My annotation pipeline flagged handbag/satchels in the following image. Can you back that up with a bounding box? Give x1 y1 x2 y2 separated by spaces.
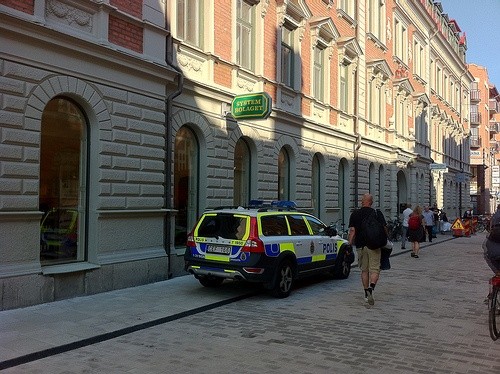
345 245 355 264
380 248 392 270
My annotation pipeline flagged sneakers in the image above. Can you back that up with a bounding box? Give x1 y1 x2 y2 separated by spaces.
366 288 374 305
365 297 368 303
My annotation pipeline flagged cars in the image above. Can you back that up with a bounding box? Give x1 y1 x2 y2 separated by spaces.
184 200 356 298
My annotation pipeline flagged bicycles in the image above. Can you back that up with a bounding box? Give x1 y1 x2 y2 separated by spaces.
476 214 492 232
329 215 402 243
484 271 500 341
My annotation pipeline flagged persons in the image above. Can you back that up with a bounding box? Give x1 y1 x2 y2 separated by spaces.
400 203 500 303
348 194 387 306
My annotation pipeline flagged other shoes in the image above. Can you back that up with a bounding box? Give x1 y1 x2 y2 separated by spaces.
401 247 406 249
411 253 419 258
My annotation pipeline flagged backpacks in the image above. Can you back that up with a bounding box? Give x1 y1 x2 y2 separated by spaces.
408 214 420 230
488 211 500 243
358 209 387 250
